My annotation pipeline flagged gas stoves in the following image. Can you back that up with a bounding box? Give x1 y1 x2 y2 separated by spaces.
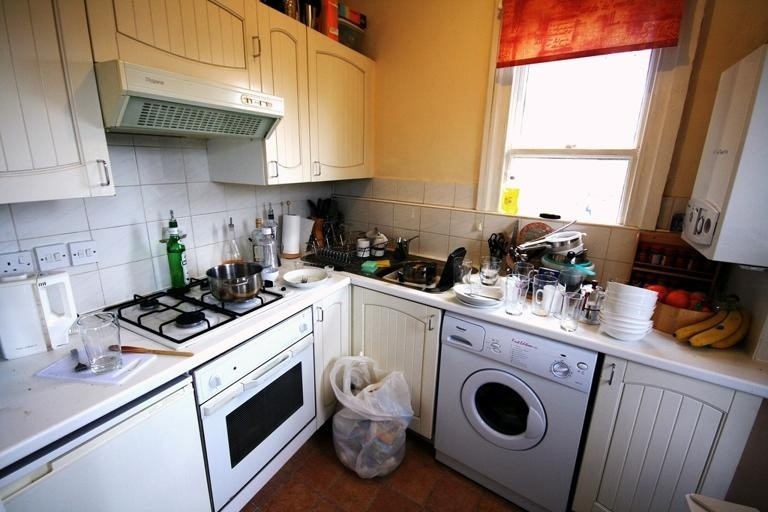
104 275 301 348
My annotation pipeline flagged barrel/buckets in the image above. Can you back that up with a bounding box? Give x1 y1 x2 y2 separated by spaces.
541 254 598 291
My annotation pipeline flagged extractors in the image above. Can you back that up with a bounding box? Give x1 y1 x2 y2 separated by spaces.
90 59 285 145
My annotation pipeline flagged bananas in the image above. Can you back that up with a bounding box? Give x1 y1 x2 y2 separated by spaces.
672 302 751 349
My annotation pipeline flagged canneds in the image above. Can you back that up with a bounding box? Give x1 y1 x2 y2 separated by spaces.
356 238 384 258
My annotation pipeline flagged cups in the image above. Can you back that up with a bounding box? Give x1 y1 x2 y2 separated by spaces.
78 311 122 373
478 256 502 284
371 236 385 256
356 238 369 257
452 255 474 282
508 260 583 331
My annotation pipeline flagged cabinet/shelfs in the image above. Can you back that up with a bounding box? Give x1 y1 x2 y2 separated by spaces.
0 1 116 206
316 284 352 430
206 1 306 186
307 25 374 186
351 279 444 445
84 1 250 88
568 352 768 511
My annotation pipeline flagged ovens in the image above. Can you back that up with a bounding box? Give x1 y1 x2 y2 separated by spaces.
199 332 317 512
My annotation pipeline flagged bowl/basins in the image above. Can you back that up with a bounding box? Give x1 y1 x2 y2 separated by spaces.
261 267 280 283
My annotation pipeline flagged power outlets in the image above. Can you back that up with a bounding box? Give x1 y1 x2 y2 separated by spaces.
0 253 20 278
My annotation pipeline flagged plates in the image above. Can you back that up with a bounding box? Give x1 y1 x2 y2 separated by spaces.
283 268 327 288
598 280 660 342
452 283 504 311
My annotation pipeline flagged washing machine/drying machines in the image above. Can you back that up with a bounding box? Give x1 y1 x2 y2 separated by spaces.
433 310 599 512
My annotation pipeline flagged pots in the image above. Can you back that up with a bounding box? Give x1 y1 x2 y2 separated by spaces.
206 260 267 304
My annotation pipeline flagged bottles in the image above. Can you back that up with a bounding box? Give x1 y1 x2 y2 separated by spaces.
249 202 281 267
165 218 192 290
224 223 242 263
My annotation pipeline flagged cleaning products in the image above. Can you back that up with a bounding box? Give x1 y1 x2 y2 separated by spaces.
500 175 521 215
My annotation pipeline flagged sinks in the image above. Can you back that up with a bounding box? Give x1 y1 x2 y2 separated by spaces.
375 260 479 293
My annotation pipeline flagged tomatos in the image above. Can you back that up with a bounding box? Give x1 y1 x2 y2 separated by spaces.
644 284 713 312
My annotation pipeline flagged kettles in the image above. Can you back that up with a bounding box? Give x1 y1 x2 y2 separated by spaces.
0 271 79 361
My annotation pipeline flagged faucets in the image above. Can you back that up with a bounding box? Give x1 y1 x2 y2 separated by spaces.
395 234 419 257
